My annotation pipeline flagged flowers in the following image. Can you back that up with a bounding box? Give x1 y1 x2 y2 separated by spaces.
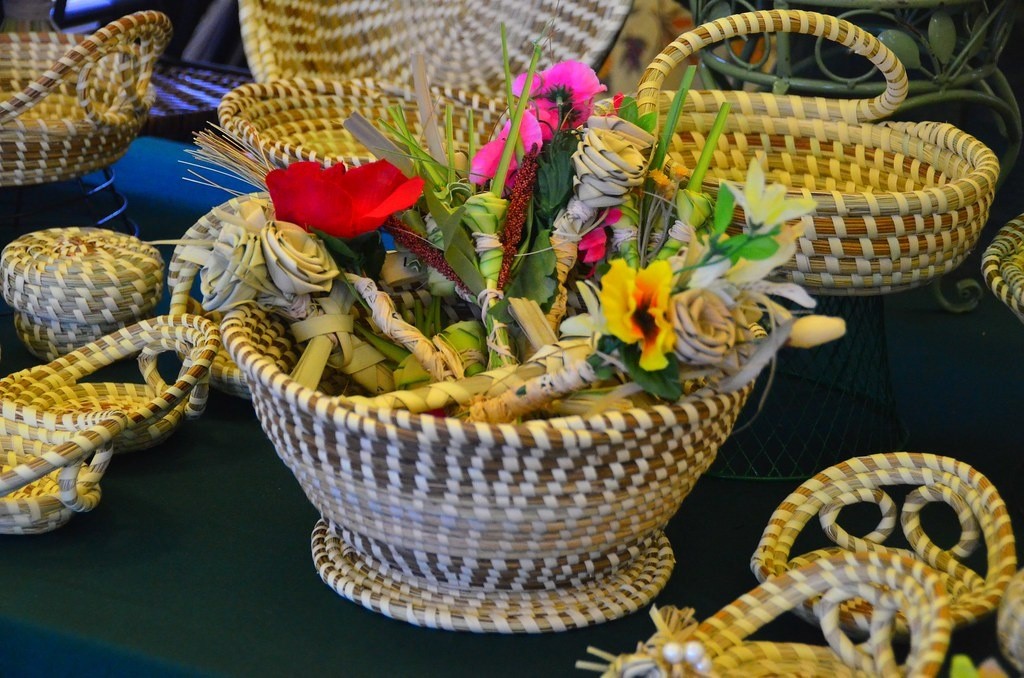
139 18 845 423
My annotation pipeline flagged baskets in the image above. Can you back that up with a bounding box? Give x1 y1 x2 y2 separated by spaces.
591 8 1001 297
0 0 770 633
981 211 1024 323
